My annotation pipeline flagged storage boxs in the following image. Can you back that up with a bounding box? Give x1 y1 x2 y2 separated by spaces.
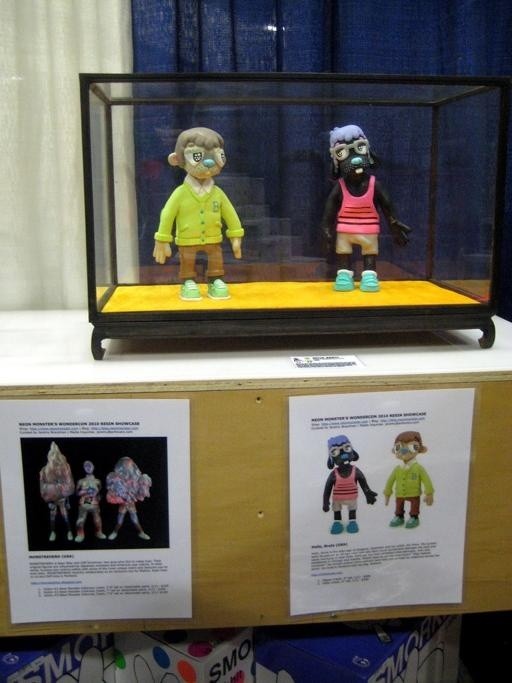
0 613 465 681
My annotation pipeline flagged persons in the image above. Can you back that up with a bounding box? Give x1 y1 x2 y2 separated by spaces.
151 127 245 300
322 125 413 293
